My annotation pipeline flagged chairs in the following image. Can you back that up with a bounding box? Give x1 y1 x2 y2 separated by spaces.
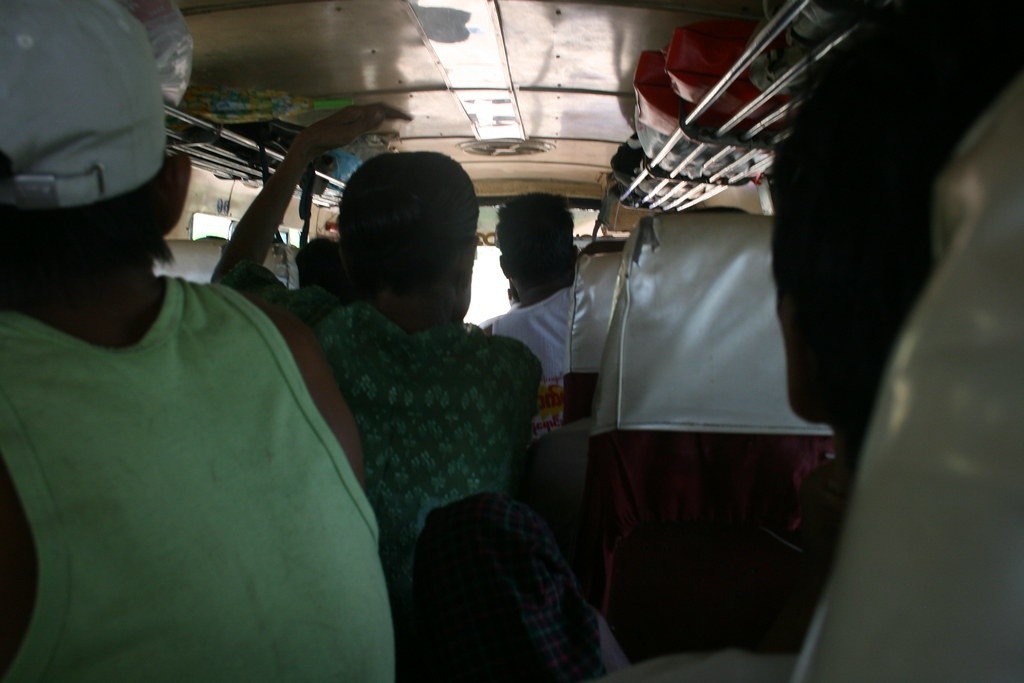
793 82 1024 683
560 250 622 426
570 212 841 664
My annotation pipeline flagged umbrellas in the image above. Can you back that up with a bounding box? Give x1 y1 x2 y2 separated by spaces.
178 70 350 124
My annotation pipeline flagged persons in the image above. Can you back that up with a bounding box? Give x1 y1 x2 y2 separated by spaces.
218 103 543 593
415 0 1023 683
297 235 341 286
0 5 396 682
492 194 578 381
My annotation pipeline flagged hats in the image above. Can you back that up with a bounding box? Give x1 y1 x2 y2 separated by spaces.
0 0 166 212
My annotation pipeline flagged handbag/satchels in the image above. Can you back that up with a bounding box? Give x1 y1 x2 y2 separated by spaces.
138 0 308 180
609 17 799 209
